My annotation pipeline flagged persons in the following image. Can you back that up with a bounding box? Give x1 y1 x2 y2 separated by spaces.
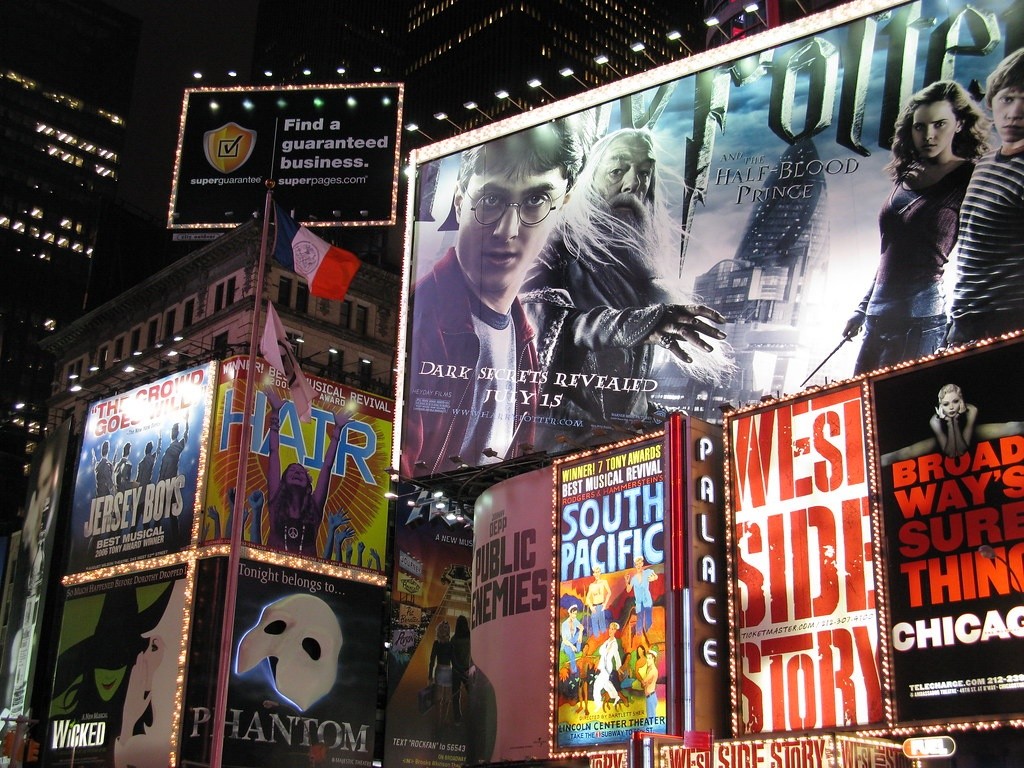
426 615 473 722
86 407 191 565
401 120 726 479
203 486 382 572
841 45 1024 377
260 383 356 558
878 384 1024 470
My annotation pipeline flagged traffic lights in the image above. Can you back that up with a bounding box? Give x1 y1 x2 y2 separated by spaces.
27 738 41 763
2 730 15 757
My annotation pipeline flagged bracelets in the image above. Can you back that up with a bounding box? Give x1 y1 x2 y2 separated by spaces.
270 417 280 432
331 435 339 441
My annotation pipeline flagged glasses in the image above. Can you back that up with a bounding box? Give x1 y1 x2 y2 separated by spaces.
461 183 569 227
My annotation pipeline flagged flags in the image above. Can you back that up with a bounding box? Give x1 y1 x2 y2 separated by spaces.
269 190 361 303
259 302 320 425
